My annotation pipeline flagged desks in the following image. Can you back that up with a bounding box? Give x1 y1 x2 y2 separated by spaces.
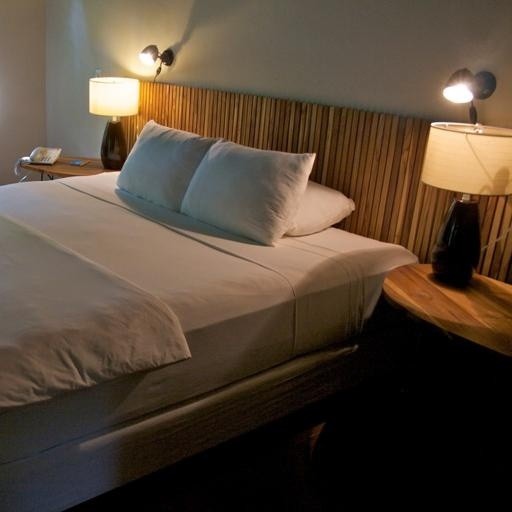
20 155 122 182
381 262 512 359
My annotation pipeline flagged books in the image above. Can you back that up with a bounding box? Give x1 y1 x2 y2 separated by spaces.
68 159 91 168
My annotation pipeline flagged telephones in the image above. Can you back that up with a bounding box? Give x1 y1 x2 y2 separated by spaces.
30 146 62 165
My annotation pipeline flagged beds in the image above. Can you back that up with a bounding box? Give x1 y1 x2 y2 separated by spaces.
0 74 512 512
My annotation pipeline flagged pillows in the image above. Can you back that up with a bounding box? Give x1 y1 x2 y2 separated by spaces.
116 119 219 214
178 136 316 250
283 178 355 238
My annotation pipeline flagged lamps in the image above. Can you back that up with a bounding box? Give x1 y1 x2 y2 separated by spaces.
138 43 174 66
445 67 496 101
420 120 512 288
88 77 140 171
